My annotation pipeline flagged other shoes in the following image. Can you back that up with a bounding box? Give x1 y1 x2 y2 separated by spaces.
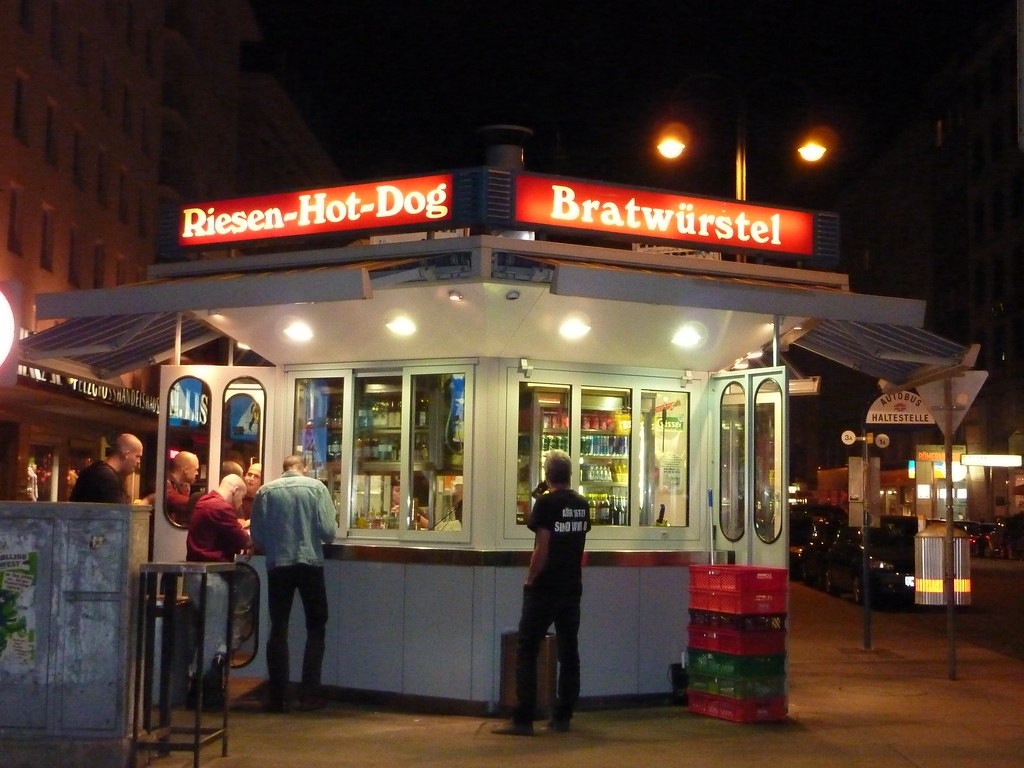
302 695 328 710
264 701 288 712
186 674 198 709
202 670 223 710
549 720 569 730
491 725 534 735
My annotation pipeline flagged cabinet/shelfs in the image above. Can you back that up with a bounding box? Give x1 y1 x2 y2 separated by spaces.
527 386 658 524
305 380 445 471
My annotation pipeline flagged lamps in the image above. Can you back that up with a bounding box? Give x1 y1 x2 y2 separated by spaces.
748 347 764 359
734 360 749 369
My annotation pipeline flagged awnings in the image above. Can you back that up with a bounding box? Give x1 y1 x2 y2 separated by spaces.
14 302 220 385
38 260 419 321
794 316 981 394
519 255 928 328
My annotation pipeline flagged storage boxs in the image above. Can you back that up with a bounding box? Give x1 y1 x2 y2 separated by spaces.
687 673 787 700
688 563 789 593
686 646 788 677
687 607 788 633
686 623 787 655
688 588 789 615
687 690 789 724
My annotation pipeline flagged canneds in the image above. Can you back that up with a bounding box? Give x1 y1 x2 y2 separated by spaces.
581 435 628 456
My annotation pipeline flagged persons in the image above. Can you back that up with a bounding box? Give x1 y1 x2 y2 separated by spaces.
220 461 244 482
69 433 155 506
491 450 592 735
391 476 429 528
249 454 337 713
152 451 199 522
236 464 261 527
181 474 256 714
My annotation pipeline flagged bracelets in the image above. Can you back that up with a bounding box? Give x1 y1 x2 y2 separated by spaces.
416 513 421 523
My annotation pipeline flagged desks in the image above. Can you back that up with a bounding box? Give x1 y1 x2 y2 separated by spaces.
134 560 238 768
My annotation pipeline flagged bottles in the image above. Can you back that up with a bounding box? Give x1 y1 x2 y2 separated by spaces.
581 436 628 456
757 502 775 534
415 435 429 461
372 401 401 428
580 460 626 482
327 409 342 428
531 480 547 499
416 399 429 428
327 435 342 461
583 494 628 526
355 410 371 436
357 508 400 529
358 439 400 461
